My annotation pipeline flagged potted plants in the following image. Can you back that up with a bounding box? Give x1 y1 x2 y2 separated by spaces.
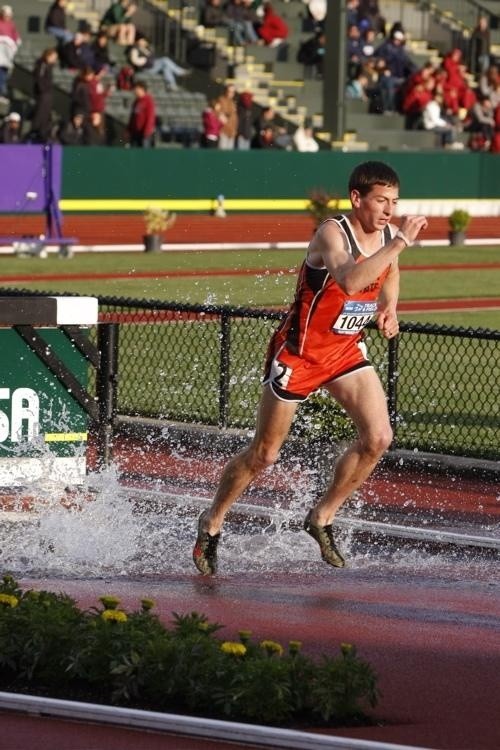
141 205 177 251
448 209 470 245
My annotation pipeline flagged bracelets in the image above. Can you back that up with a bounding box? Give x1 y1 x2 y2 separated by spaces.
396 230 410 247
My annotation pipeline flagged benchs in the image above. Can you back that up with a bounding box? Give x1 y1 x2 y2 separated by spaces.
0 0 499 150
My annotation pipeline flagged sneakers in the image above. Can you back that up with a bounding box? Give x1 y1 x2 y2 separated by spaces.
303 510 346 568
193 509 220 577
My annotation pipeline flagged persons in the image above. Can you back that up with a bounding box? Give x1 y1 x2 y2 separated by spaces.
193 161 429 576
0 1 320 152
295 1 500 152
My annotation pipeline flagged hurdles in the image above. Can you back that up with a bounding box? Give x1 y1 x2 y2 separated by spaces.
0 296 119 493
